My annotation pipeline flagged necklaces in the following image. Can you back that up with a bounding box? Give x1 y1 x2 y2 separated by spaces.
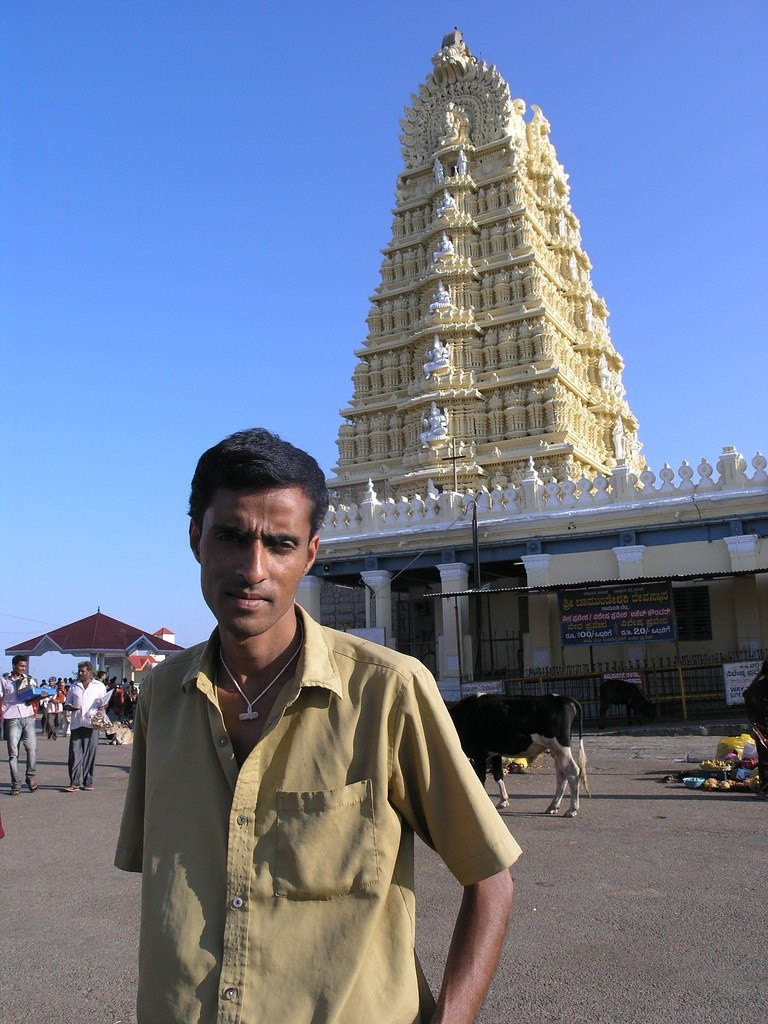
220 617 303 720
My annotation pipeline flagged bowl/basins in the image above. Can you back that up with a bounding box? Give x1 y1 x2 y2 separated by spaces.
705 771 731 780
683 777 705 788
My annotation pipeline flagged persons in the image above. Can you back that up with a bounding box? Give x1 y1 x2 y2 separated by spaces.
551 164 638 460
433 236 455 261
743 660 768 800
437 192 456 217
423 334 451 379
512 98 528 150
429 283 451 314
420 403 449 449
0 655 144 795
113 427 523 1024
433 158 444 182
456 150 467 175
437 110 460 147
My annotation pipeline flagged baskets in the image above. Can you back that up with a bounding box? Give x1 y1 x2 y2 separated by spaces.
683 777 705 789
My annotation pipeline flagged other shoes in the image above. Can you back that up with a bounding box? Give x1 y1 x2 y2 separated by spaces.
53 733 56 740
10 788 20 795
63 784 80 791
26 778 39 792
48 734 51 739
760 791 768 800
84 785 94 790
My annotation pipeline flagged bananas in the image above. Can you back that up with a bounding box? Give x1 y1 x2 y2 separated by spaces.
700 758 735 767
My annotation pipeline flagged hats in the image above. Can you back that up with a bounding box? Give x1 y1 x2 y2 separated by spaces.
48 676 56 682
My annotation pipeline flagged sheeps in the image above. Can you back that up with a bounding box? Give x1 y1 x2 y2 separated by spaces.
105 721 134 746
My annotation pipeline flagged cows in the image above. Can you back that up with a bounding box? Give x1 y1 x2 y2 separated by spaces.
443 692 591 818
597 679 657 733
